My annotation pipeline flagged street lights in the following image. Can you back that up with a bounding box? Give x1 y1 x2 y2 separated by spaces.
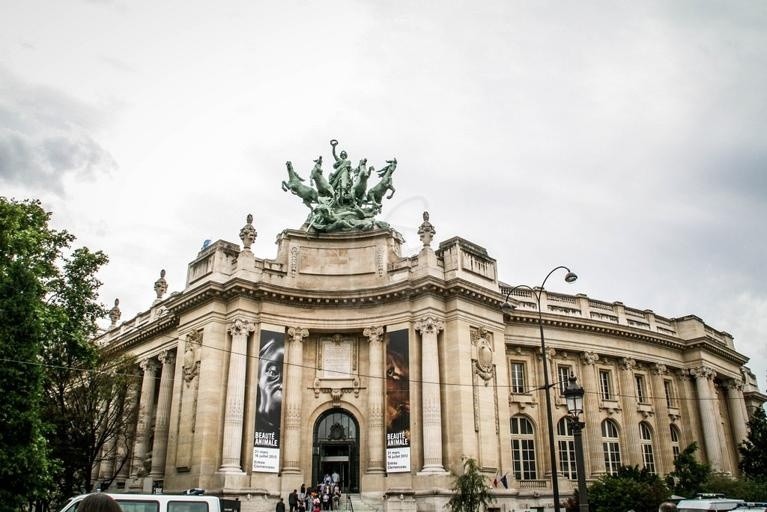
501 266 589 512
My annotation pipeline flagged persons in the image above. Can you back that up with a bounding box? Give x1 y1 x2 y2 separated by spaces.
239 213 257 249
386 343 410 431
258 338 284 427
328 139 354 195
275 471 341 512
154 269 168 298
108 298 121 325
417 210 436 246
76 493 123 512
305 201 381 233
658 501 678 512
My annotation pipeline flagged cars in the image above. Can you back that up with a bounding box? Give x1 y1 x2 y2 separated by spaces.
676 492 767 512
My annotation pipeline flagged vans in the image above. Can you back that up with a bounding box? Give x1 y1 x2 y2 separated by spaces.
54 493 221 512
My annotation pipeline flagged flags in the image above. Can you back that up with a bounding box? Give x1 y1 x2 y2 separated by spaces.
501 475 508 489
